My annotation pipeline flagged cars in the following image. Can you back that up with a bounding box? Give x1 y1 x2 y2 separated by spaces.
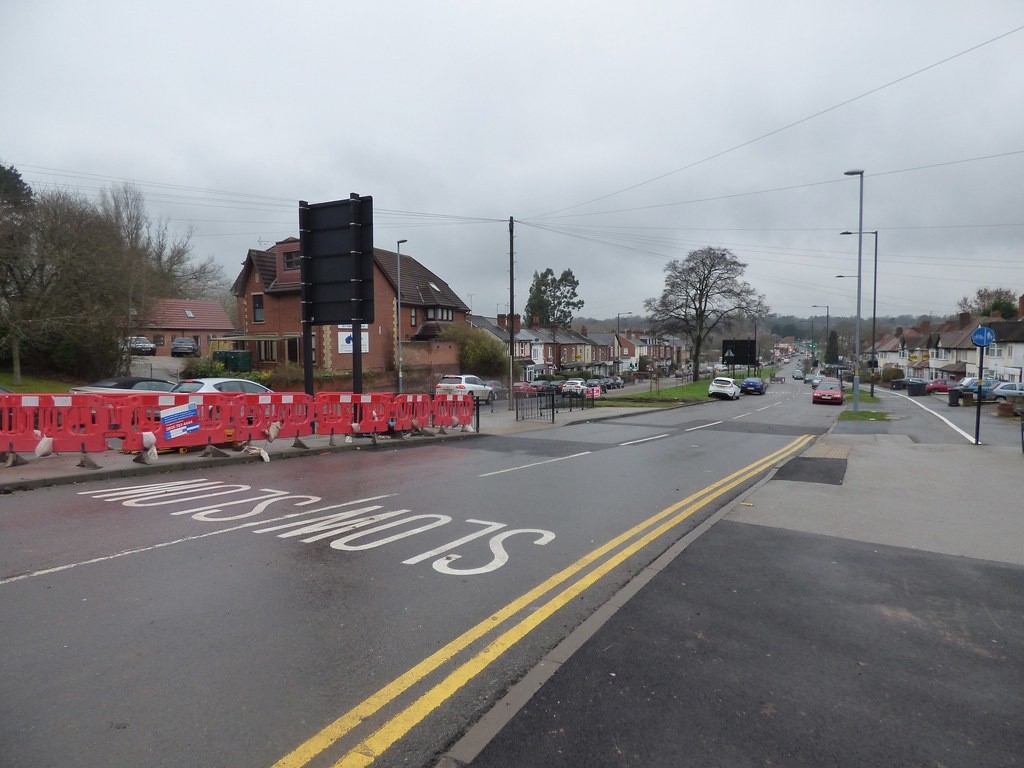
123 336 156 356
741 377 766 395
812 380 845 405
708 377 741 400
562 380 586 398
925 379 959 395
68 376 176 440
568 378 584 382
531 381 550 396
986 381 1024 402
586 379 607 394
958 377 1000 395
513 382 538 398
0 386 40 430
793 371 804 380
171 378 275 424
485 381 509 401
706 350 805 373
904 378 927 386
550 380 567 394
811 376 826 389
171 337 199 357
603 376 624 389
804 374 814 383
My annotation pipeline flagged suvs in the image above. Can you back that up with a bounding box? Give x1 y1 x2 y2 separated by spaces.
436 375 494 405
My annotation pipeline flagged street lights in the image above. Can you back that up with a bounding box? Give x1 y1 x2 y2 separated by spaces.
812 305 829 343
801 320 813 351
617 311 633 372
843 168 865 414
836 230 878 398
396 240 408 394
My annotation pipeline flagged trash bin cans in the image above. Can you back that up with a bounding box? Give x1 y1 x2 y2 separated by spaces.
214 349 251 373
890 380 909 390
948 388 960 406
907 381 926 396
